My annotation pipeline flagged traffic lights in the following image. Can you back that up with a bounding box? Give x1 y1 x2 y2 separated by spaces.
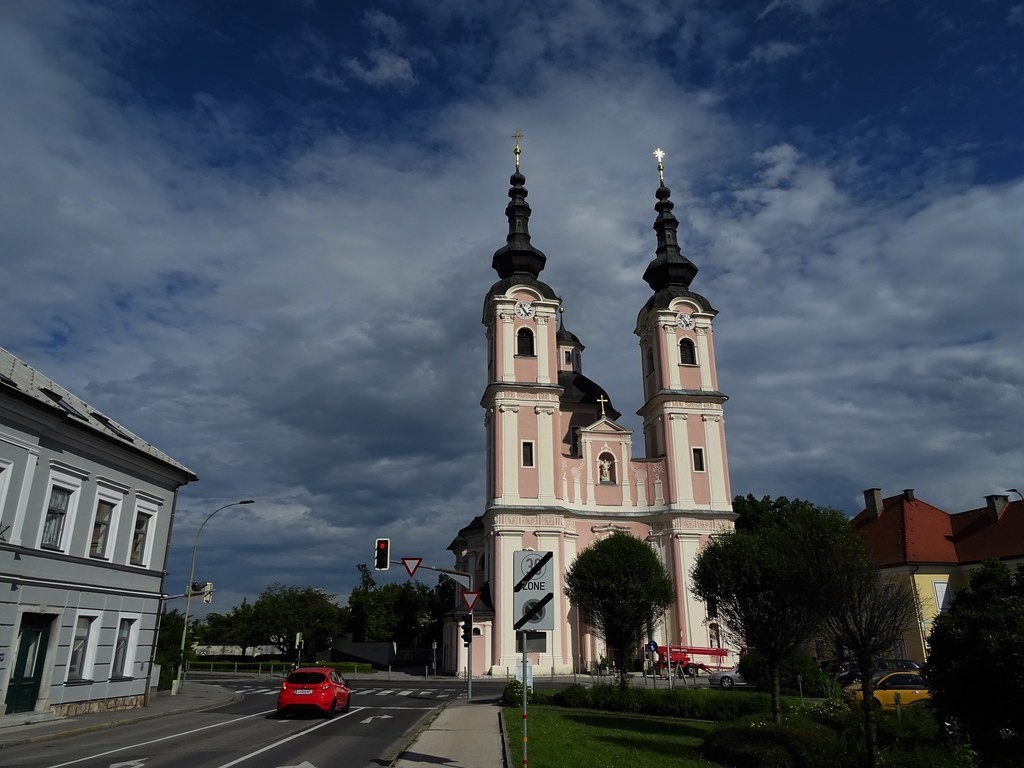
473 627 481 637
461 614 473 647
374 538 389 570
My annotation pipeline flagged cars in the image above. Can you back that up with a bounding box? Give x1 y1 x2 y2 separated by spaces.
278 666 352 720
834 659 922 689
708 663 751 687
840 670 933 711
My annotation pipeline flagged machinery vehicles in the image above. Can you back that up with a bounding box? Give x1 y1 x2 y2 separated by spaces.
642 643 730 681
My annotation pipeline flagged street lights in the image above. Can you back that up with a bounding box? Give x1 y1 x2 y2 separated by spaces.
173 498 256 694
